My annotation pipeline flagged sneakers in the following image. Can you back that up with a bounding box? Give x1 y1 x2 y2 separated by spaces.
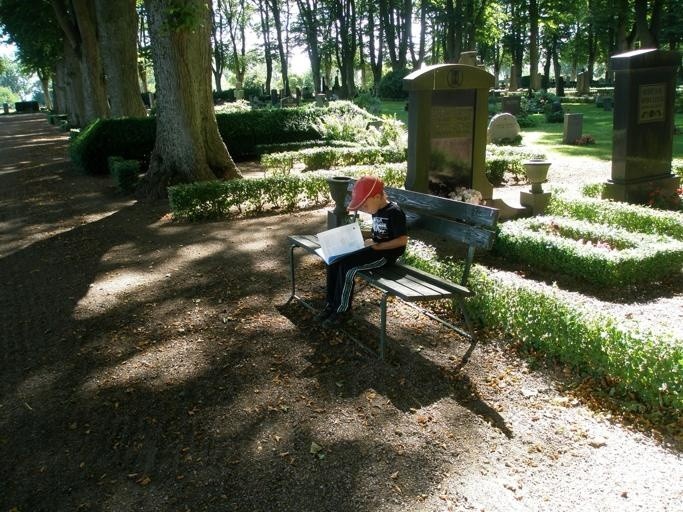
322 309 353 330
312 302 334 322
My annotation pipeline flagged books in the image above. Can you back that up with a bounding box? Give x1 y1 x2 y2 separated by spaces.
315 222 377 266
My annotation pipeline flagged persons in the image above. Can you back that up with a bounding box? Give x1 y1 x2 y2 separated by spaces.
313 176 408 330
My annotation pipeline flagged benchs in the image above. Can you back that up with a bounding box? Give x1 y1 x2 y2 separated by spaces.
280 178 499 363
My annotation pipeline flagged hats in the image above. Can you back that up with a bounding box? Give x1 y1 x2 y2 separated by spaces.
346 173 385 212
